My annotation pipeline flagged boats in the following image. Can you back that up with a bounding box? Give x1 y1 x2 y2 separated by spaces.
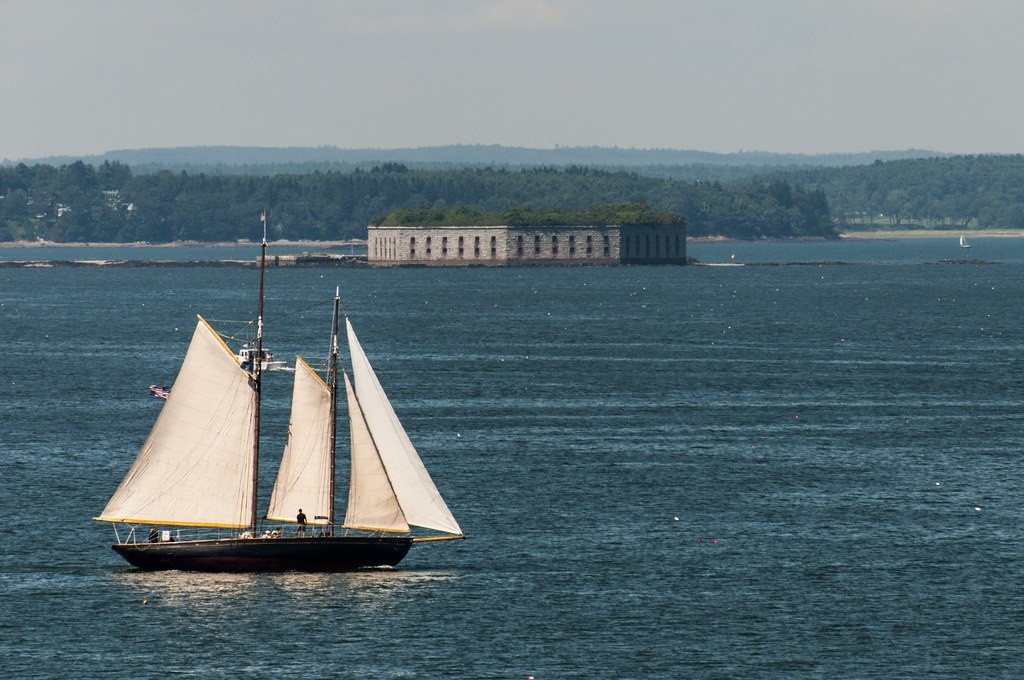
232 347 286 371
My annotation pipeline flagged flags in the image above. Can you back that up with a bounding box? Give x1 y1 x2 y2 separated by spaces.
148 385 171 399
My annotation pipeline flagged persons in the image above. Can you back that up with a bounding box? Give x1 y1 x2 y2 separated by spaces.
149 509 307 542
265 352 273 361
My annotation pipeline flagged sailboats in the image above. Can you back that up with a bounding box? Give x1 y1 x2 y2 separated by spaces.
89 213 468 572
